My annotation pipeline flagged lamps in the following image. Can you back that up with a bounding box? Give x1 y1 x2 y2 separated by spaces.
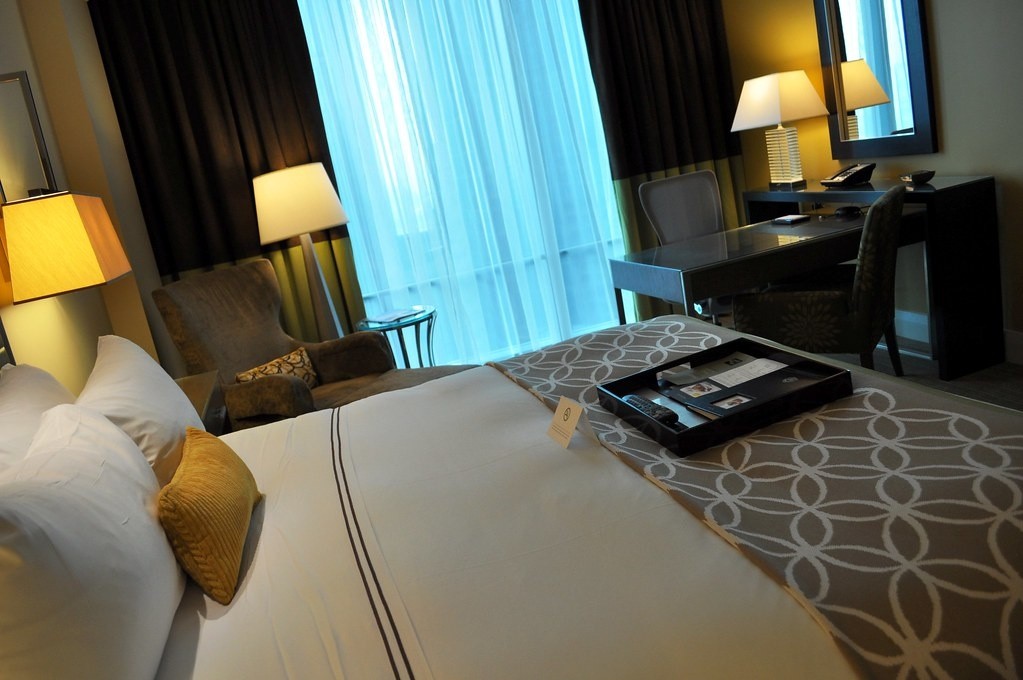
839 58 890 138
253 163 349 342
731 70 830 192
0 188 132 304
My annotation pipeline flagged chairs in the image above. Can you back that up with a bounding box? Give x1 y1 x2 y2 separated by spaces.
638 170 771 328
730 186 903 377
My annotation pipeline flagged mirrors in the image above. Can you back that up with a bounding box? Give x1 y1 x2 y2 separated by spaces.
0 70 58 203
812 0 939 160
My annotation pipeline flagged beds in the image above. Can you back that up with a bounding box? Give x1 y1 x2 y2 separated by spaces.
0 316 1021 680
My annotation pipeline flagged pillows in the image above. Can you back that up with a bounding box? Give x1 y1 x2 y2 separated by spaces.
236 346 317 392
0 404 190 680
75 334 206 466
156 426 265 604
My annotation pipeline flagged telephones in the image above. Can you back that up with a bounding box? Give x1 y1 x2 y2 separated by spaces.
820 162 877 189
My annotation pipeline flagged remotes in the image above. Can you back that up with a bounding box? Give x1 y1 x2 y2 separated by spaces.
622 395 679 426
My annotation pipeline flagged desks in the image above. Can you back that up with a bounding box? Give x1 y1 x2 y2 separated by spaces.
609 205 925 326
356 305 437 370
735 174 1005 383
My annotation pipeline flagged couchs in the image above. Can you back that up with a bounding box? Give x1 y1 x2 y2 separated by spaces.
153 254 479 432
